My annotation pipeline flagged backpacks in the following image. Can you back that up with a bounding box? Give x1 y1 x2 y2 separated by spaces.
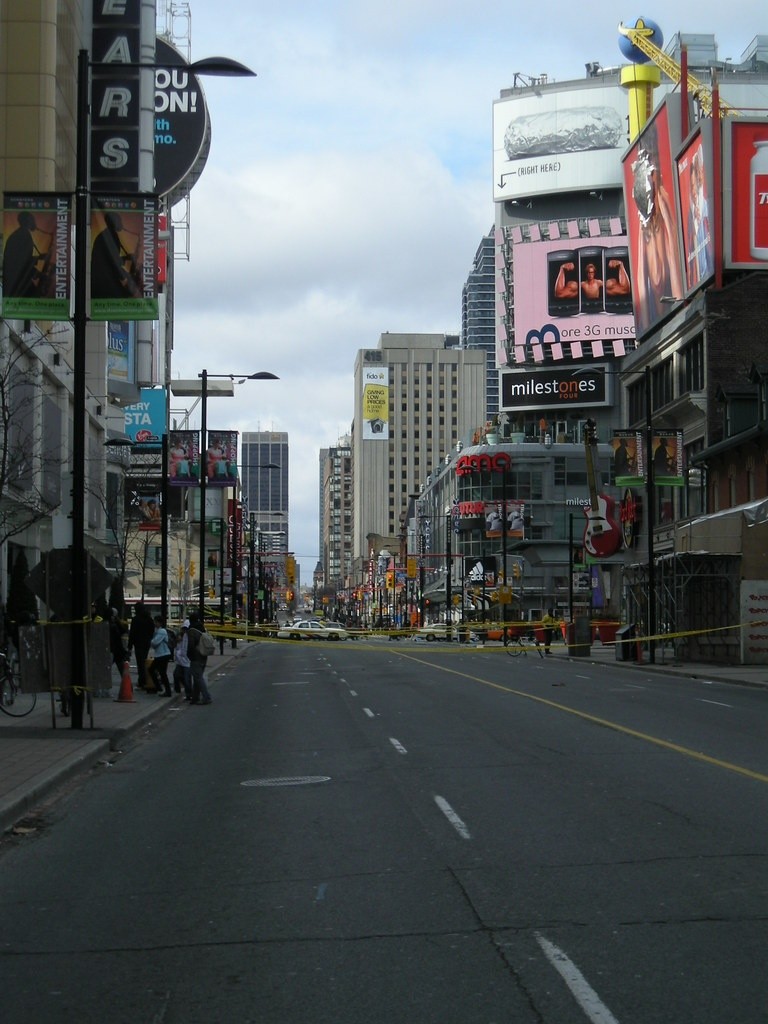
188 627 215 656
163 628 177 649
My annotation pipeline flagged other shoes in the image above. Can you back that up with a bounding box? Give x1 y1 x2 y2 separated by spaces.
197 695 213 705
190 698 201 705
183 696 192 700
134 684 148 691
158 690 171 697
151 684 163 692
171 687 181 694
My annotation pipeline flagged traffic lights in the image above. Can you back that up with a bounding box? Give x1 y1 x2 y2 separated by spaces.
353 592 357 600
253 600 257 606
287 590 293 604
386 572 394 589
423 599 430 606
190 560 196 577
288 575 295 584
239 593 248 607
513 562 519 579
498 570 503 584
210 589 216 599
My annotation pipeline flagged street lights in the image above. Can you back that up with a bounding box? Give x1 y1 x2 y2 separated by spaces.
459 464 509 649
571 365 658 663
230 463 282 650
383 544 408 627
246 511 284 631
396 533 425 628
417 514 452 641
196 368 280 621
70 44 257 733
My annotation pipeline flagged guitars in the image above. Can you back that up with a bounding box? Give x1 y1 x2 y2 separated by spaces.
582 417 624 559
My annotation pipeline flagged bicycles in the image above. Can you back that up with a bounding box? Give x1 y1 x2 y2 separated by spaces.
0 647 37 717
505 634 545 659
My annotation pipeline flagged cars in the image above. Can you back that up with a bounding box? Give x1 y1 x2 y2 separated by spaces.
277 620 347 642
305 609 311 614
278 605 287 611
417 622 512 645
293 616 303 624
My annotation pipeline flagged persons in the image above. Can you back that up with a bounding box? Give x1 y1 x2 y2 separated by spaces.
148 615 171 697
127 600 155 689
206 440 228 478
109 608 134 693
167 438 193 477
541 608 554 654
139 499 161 523
172 613 213 705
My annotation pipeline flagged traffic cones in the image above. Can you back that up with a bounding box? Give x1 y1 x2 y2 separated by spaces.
114 661 138 702
